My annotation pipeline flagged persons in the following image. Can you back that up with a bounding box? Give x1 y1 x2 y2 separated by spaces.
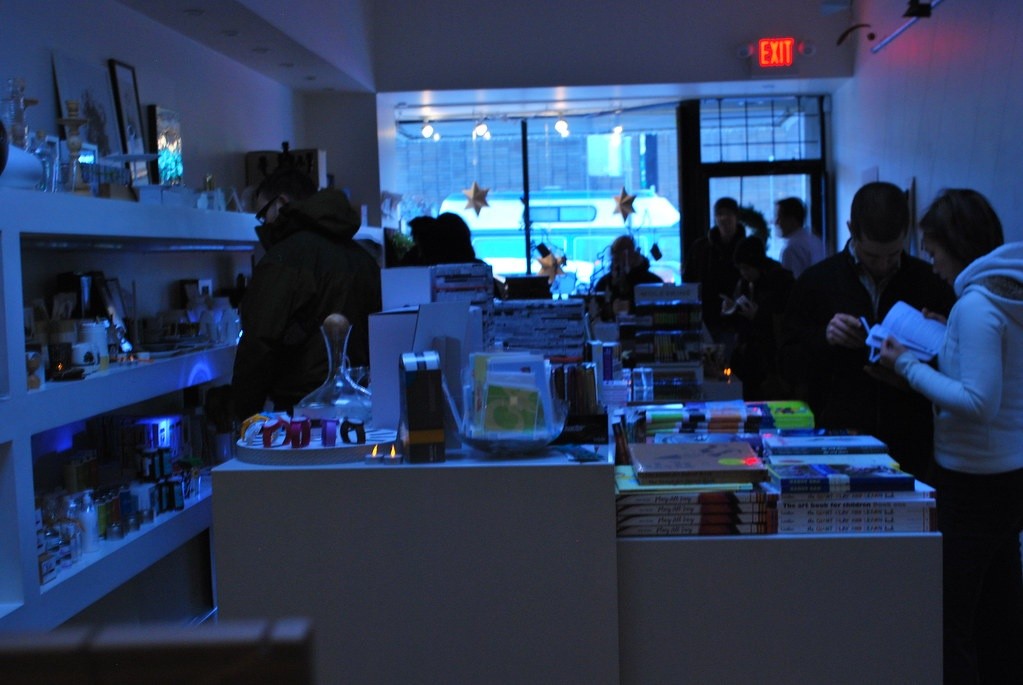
778 181 958 470
233 166 382 413
402 213 502 296
881 189 1023 685
776 198 828 279
593 236 662 324
723 235 794 400
687 198 768 361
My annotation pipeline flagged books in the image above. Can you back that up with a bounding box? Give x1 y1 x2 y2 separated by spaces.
865 301 948 361
435 264 936 536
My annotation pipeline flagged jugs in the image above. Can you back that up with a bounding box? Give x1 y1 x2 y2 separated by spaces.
297 314 372 426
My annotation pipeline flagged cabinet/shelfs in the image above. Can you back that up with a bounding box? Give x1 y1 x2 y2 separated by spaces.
0 187 383 637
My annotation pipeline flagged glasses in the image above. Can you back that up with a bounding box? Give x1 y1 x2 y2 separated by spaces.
255 194 280 225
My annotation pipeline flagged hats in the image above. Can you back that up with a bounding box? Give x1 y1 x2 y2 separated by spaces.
714 198 739 215
732 237 765 266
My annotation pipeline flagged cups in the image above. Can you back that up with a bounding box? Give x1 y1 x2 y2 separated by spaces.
80 324 109 370
71 343 94 375
90 344 102 373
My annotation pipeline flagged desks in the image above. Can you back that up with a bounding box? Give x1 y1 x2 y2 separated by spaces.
618 532 943 684
209 441 619 684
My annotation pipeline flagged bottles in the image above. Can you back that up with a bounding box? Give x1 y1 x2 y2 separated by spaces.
28 130 57 193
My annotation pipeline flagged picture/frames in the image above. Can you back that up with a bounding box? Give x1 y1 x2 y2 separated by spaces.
109 59 150 182
